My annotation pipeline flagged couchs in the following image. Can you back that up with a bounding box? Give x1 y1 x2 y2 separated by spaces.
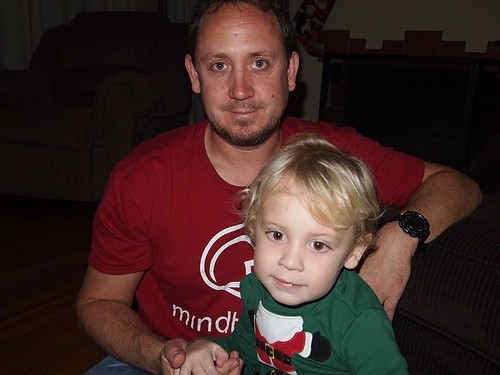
358 189 500 375
1 10 194 206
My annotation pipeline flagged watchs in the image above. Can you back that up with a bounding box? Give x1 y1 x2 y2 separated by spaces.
384 211 432 252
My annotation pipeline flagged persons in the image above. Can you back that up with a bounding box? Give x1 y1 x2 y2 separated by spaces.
173 130 411 375
74 0 482 375
